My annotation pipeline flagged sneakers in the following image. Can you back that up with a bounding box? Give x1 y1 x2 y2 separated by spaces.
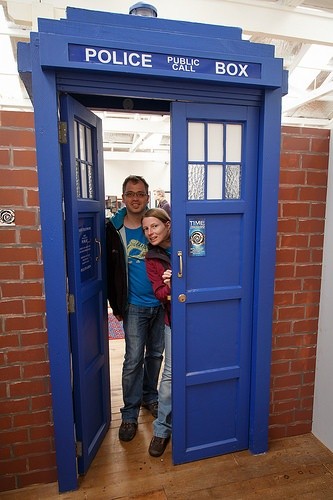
119 421 138 441
149 436 171 457
142 401 171 422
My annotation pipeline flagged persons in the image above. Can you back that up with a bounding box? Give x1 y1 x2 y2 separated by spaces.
152 189 170 217
104 175 164 441
141 208 194 457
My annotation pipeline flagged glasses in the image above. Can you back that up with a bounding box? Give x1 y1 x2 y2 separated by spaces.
123 192 148 197
